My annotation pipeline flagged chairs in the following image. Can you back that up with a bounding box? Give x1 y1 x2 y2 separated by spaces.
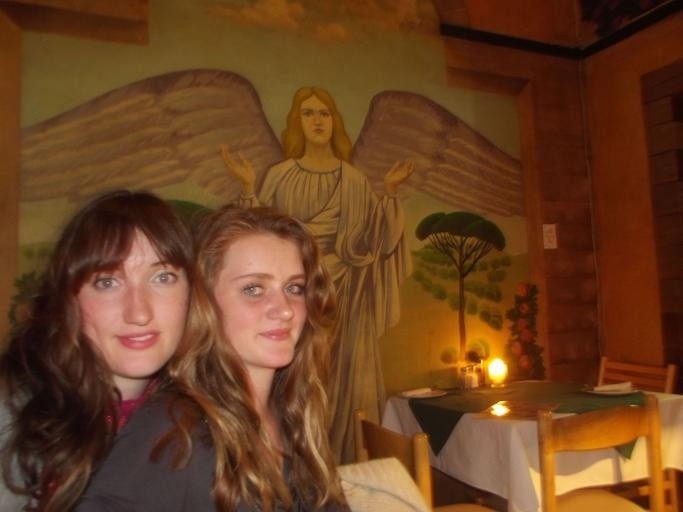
538 356 681 511
353 408 494 512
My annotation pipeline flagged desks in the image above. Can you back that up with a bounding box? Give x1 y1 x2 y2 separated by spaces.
390 380 682 512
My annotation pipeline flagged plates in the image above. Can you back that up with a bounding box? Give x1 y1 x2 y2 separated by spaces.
588 387 642 396
397 388 447 399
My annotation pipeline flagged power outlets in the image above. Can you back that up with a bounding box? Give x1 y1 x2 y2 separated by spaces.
543 224 558 250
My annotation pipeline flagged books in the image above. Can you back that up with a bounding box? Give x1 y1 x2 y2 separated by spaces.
472 400 561 420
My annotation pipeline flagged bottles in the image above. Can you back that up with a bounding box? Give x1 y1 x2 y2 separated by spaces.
459 366 479 390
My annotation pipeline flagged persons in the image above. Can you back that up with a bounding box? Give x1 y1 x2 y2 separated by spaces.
1 189 200 512
221 87 417 467
71 202 353 512
